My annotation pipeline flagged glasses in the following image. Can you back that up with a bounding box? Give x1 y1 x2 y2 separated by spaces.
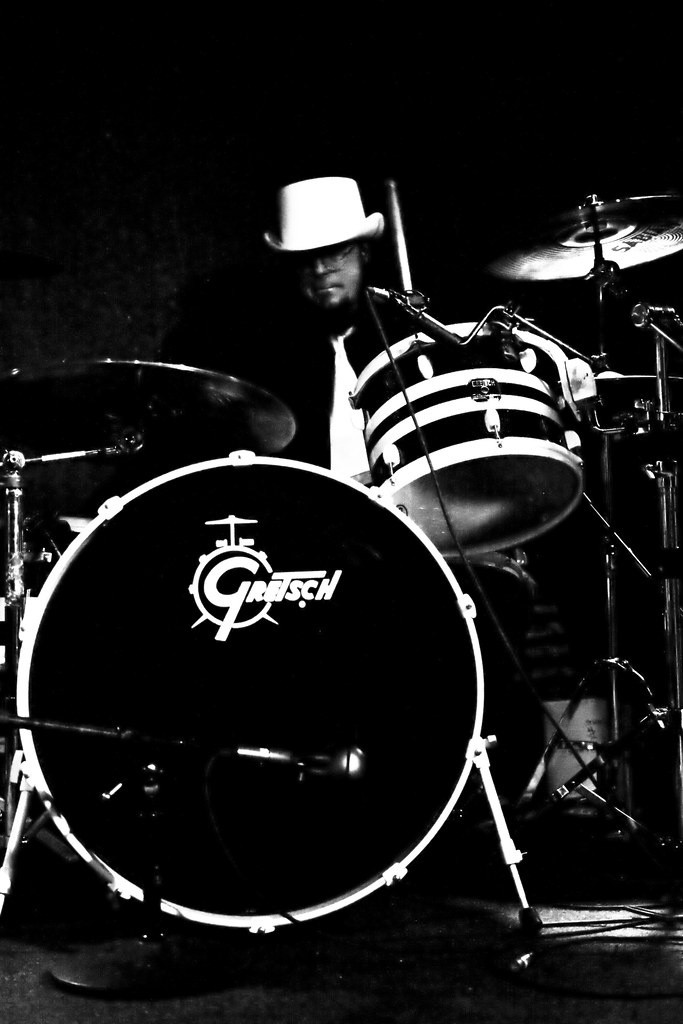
297 245 357 274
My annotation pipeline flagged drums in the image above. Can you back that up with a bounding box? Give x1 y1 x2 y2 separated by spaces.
352 319 587 559
14 445 488 934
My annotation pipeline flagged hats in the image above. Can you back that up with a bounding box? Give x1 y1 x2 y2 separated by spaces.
265 176 385 251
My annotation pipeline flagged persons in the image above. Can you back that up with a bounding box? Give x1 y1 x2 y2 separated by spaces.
228 177 421 488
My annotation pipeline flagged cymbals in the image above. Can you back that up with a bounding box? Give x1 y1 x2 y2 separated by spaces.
0 357 298 462
593 369 683 389
473 191 683 284
